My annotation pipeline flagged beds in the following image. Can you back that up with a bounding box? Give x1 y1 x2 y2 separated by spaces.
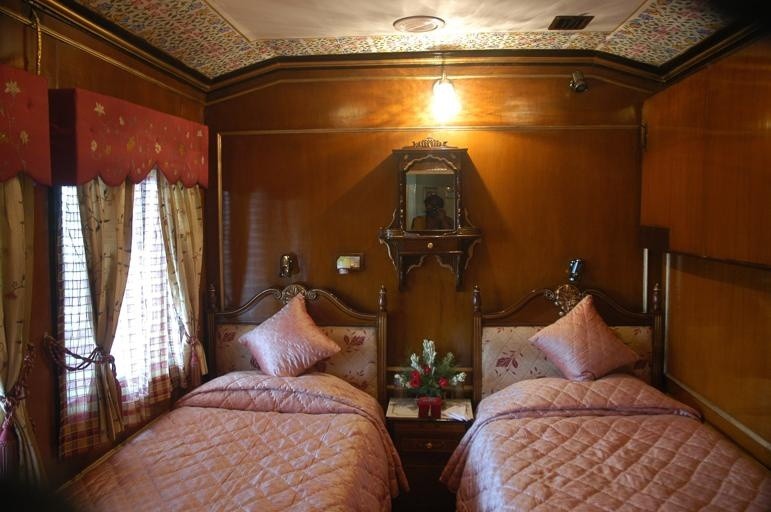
50 282 410 511
438 281 771 512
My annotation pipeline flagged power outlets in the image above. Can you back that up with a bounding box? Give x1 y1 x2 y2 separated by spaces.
336 256 361 268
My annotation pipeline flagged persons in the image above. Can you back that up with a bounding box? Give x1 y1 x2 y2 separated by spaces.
411 189 452 228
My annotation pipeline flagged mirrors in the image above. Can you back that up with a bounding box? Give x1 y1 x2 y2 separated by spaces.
388 138 474 235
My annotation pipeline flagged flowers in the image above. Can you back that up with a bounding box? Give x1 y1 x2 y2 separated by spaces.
392 339 467 397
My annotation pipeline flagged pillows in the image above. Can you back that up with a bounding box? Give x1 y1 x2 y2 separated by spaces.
238 292 341 377
527 294 640 382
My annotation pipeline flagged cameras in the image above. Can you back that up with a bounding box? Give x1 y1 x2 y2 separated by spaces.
426 206 438 216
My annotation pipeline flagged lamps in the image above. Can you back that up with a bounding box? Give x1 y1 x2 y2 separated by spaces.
568 259 584 282
279 254 292 279
569 69 587 92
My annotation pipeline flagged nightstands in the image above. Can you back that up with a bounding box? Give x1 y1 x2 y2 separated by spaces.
385 397 474 512
